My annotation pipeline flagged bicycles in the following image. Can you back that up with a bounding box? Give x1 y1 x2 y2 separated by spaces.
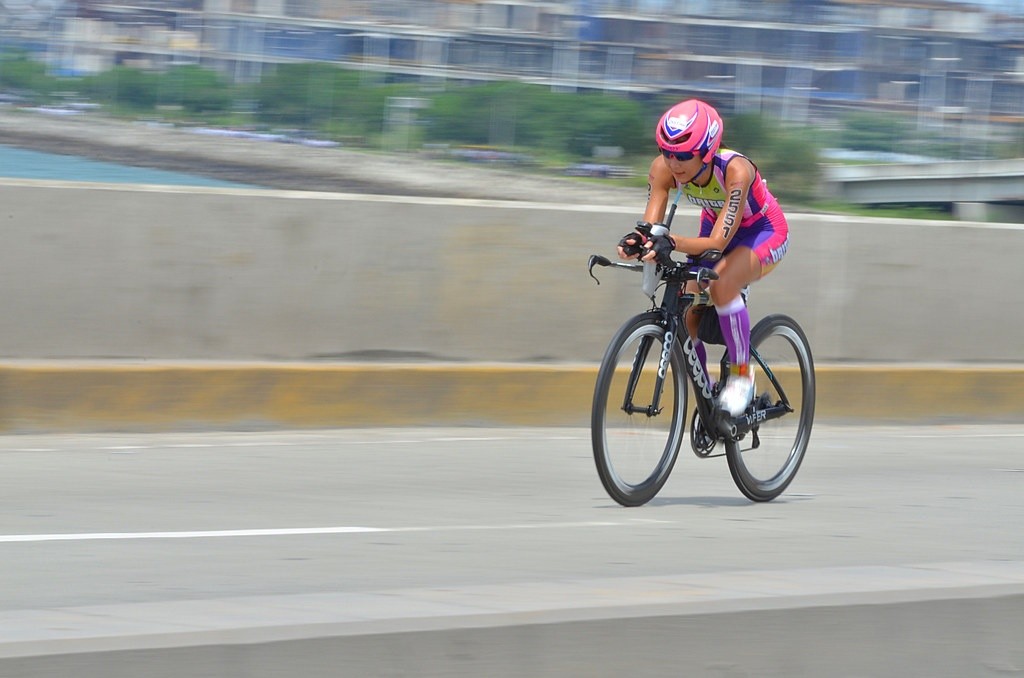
585 246 815 507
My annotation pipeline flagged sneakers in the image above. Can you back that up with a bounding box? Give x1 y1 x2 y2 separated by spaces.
717 361 756 417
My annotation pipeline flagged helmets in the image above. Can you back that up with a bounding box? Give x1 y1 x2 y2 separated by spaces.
654 97 724 185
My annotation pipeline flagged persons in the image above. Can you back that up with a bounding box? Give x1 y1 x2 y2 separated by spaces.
616 99 789 442
